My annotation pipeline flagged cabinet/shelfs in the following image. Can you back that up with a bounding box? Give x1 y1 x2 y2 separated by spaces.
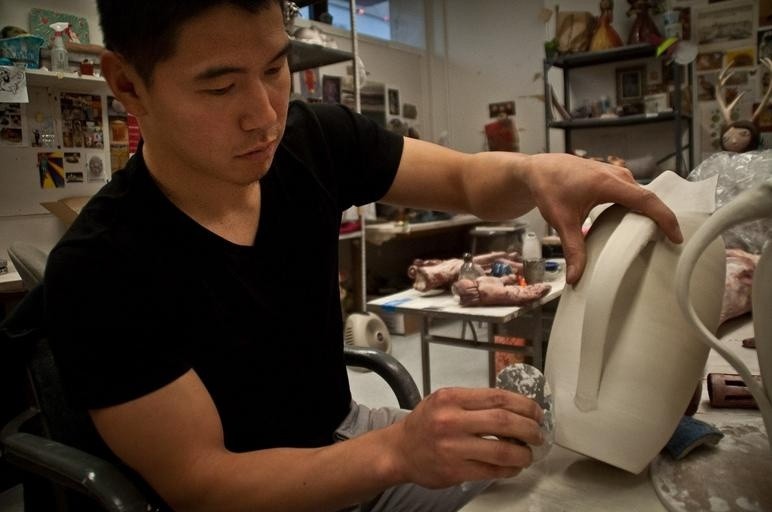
290 0 368 313
541 41 692 184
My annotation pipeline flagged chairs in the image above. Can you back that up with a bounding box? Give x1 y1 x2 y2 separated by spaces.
0 287 423 510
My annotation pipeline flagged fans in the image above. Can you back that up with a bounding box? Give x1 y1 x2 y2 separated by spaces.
343 314 393 372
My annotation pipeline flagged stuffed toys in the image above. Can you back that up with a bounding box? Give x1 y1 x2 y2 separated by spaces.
719 120 759 152
625 0 667 45
589 0 622 53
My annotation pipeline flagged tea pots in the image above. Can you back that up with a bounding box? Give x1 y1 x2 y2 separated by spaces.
674 174 772 451
543 202 728 477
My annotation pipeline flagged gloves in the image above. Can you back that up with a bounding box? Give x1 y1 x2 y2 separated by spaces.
413 259 465 292
451 274 551 308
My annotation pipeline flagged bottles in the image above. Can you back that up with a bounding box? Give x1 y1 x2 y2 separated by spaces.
523 232 542 278
459 252 476 283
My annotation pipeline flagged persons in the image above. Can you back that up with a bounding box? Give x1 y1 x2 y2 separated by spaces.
45 0 683 510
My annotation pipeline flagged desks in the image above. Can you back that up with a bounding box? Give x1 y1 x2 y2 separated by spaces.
368 258 566 398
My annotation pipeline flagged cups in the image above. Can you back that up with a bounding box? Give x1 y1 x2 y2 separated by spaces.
523 258 545 285
